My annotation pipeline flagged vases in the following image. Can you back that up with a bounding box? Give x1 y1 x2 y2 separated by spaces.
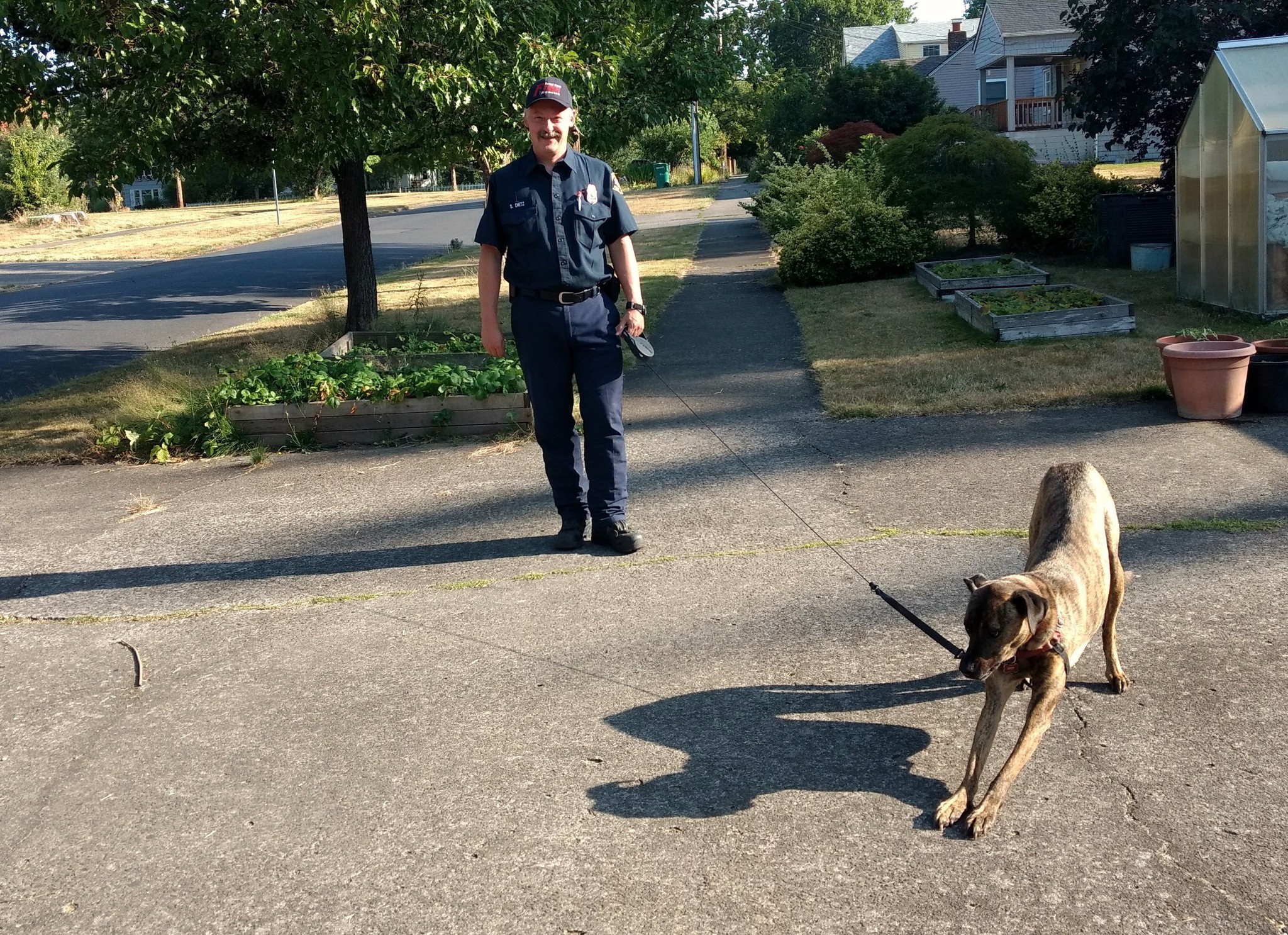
1251 338 1288 356
1249 353 1288 413
1163 341 1257 420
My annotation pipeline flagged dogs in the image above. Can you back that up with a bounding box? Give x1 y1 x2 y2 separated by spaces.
932 460 1130 842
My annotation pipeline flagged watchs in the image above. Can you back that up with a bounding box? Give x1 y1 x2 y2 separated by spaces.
624 300 647 317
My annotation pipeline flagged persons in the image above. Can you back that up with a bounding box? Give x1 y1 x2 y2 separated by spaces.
474 77 646 554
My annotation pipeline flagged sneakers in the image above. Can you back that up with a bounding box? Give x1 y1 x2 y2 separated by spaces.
591 520 646 553
555 518 592 550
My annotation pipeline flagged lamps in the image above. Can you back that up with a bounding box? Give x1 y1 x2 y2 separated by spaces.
1044 57 1053 62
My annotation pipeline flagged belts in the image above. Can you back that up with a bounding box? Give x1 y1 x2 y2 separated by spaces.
510 285 601 304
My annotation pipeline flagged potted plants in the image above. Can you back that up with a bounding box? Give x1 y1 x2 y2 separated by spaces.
213 330 533 446
1155 327 1244 398
915 255 1051 300
955 283 1136 342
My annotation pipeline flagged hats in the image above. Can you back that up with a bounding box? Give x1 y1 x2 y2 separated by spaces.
526 77 573 110
